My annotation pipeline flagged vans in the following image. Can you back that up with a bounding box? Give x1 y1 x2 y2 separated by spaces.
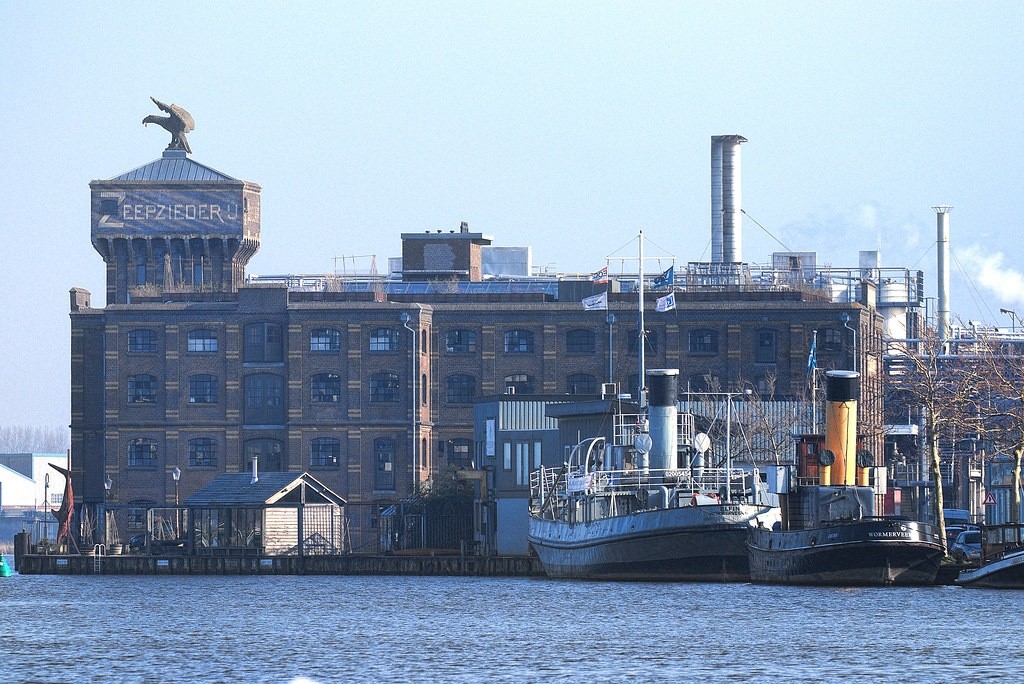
943 508 970 526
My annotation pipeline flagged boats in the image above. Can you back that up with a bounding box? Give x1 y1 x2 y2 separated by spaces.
957 521 1024 590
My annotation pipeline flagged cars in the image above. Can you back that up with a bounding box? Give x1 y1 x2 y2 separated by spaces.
945 527 963 554
950 524 980 532
950 531 982 564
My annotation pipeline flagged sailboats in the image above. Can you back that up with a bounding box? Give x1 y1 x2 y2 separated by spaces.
527 231 782 586
746 332 946 587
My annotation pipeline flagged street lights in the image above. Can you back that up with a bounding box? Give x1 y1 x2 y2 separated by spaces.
173 467 181 539
104 474 113 547
